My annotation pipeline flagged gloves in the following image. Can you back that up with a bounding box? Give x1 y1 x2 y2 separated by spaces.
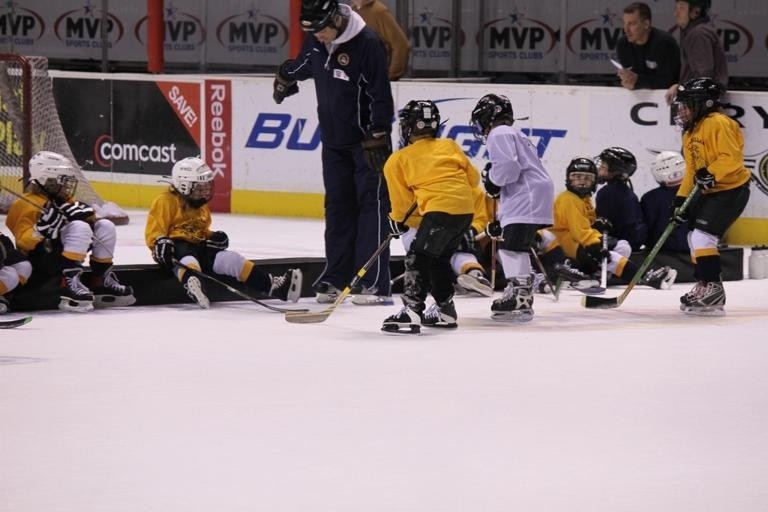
361 132 390 172
584 243 611 266
34 201 67 240
592 216 614 234
59 201 96 221
151 236 178 270
484 220 502 239
272 64 299 104
667 196 691 225
388 219 409 239
693 167 718 190
201 230 228 251
484 172 500 199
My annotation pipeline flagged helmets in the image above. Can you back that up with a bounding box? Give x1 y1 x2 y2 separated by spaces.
593 145 638 186
675 1 711 22
299 0 339 33
670 77 720 130
650 150 685 187
468 93 515 145
170 157 216 207
28 150 80 204
398 99 441 138
565 157 598 198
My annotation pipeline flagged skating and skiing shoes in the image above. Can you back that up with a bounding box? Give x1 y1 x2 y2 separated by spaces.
679 275 726 307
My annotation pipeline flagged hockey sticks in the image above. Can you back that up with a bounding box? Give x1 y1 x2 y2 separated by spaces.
0 185 115 258
172 258 308 314
0 317 32 328
571 226 609 294
581 183 698 308
286 203 418 325
532 248 564 300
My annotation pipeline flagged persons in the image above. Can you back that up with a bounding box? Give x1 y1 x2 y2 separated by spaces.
273 0 395 296
145 157 294 307
665 0 729 105
5 150 134 301
616 2 681 90
0 223 33 315
351 0 409 80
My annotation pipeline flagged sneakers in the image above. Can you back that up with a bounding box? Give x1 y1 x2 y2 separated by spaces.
311 281 340 294
491 281 534 312
349 279 391 296
421 298 457 325
534 274 552 294
645 265 671 289
185 276 210 308
382 301 422 327
58 268 95 303
88 270 135 297
456 273 493 296
271 268 294 302
